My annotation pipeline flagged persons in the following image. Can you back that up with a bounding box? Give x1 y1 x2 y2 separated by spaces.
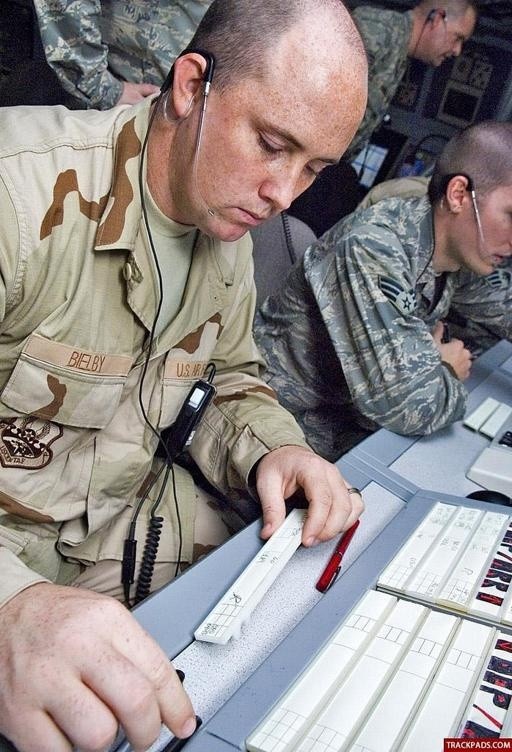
286 0 477 238
252 118 512 465
1 1 369 752
33 1 214 111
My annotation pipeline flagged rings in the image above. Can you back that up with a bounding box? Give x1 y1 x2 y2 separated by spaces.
348 488 361 496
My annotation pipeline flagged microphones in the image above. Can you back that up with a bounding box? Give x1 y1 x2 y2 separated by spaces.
192 50 216 217
442 14 453 41
471 186 501 267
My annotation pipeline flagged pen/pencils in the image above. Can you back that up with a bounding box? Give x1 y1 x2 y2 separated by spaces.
113 669 203 752
316 520 360 594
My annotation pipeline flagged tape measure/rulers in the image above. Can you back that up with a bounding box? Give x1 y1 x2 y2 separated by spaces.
193 508 308 645
